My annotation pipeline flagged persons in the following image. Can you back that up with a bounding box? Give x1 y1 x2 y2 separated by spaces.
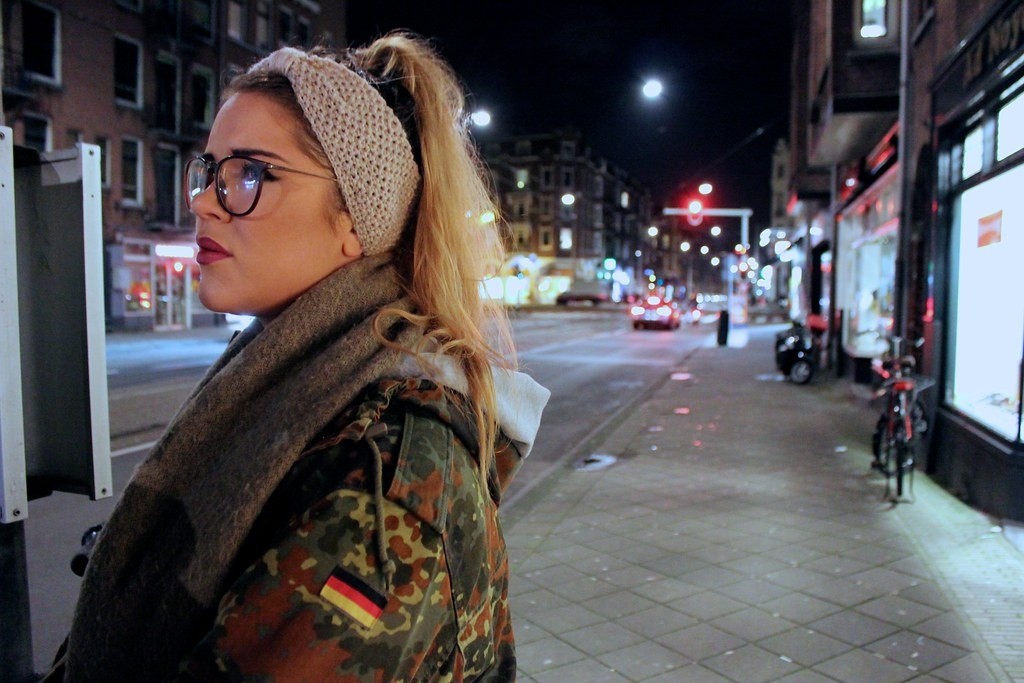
46 32 552 683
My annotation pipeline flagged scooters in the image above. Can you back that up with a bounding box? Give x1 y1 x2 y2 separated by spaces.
776 311 829 387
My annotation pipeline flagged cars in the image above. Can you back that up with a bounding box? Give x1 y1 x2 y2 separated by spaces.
631 292 682 332
557 276 727 322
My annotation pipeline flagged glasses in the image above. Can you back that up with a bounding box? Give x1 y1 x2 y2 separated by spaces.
181 156 355 216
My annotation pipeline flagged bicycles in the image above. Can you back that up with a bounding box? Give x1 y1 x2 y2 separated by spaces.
859 324 936 506
